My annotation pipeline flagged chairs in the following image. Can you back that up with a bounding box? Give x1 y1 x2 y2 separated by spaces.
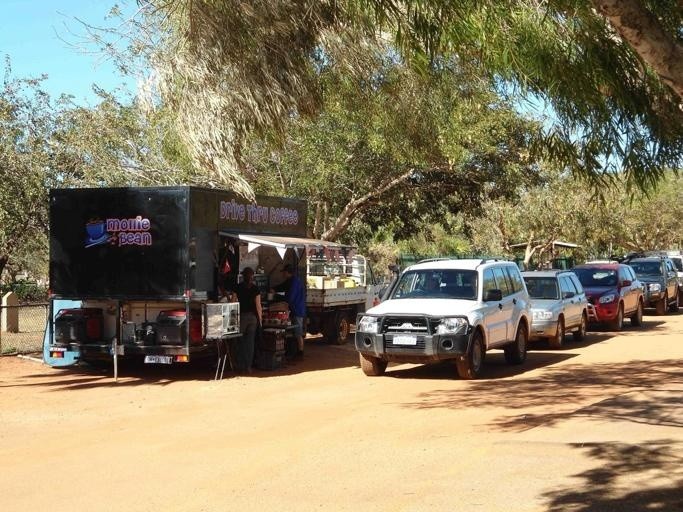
458 276 476 298
440 274 459 296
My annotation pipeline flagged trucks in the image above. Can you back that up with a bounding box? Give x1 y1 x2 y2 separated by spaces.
305 255 385 344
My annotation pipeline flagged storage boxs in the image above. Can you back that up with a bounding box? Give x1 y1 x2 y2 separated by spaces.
324 279 355 289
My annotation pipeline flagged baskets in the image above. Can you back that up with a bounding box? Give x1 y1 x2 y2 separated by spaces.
253 332 285 370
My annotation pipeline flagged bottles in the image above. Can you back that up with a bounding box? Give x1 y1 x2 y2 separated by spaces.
256 264 265 274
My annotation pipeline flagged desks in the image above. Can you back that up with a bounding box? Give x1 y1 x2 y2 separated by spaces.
204 333 245 380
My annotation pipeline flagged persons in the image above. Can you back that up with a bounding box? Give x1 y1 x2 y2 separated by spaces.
270 264 306 357
230 267 263 373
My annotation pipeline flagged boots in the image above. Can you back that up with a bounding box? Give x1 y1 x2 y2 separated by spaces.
286 348 304 362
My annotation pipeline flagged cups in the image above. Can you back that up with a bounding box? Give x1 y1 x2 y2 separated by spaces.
85 221 108 240
266 292 275 301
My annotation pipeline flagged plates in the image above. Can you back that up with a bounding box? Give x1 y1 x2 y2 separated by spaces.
84 234 107 244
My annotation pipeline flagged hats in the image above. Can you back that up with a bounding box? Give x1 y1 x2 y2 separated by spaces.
280 264 294 272
